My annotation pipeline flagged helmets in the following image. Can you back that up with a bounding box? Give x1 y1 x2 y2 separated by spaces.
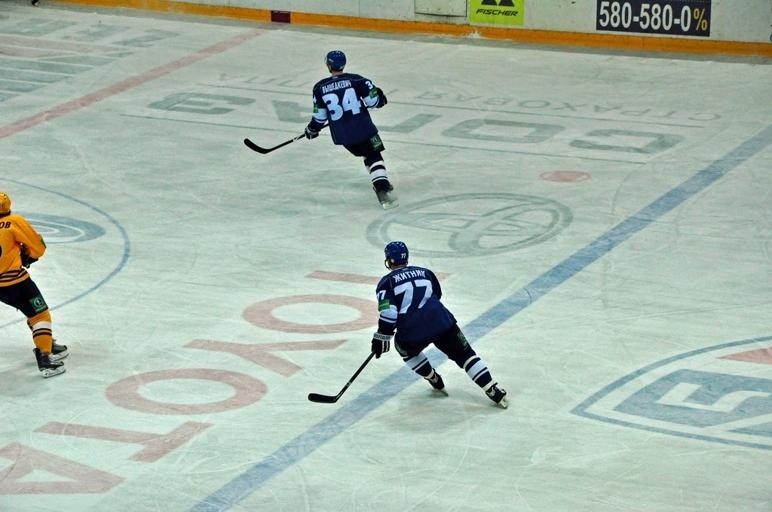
0 193 12 215
303 125 321 139
385 242 409 268
322 49 346 71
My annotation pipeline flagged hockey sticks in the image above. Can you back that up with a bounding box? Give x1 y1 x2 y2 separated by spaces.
308 353 375 403
244 124 330 154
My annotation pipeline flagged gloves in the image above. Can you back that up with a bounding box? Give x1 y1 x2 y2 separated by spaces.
371 332 395 358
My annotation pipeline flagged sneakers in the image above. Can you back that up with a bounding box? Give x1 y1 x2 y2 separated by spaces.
33 348 64 371
424 368 444 389
48 340 69 360
372 179 399 205
485 382 506 403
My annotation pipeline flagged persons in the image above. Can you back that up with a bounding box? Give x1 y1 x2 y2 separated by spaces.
0 192 68 372
304 50 398 203
372 241 507 405
